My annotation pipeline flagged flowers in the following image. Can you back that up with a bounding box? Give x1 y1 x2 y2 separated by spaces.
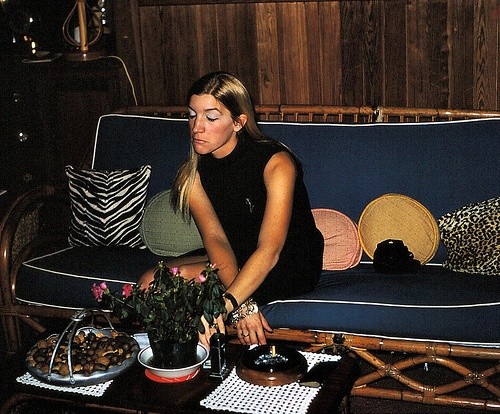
93 261 220 335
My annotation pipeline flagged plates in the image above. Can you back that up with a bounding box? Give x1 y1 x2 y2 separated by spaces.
26 327 139 387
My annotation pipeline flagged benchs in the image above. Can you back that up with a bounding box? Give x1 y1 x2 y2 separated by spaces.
1 105 500 410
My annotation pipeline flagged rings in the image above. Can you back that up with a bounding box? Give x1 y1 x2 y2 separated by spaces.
241 333 250 337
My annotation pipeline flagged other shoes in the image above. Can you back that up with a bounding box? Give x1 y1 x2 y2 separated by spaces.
372 240 426 277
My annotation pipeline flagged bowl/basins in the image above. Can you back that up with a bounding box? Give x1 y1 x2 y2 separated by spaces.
138 344 209 378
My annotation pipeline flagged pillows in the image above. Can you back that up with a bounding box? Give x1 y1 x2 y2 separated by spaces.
437 197 500 275
140 188 205 257
358 193 439 267
311 209 363 270
64 164 151 250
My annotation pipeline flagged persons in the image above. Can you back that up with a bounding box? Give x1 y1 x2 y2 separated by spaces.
134 71 324 346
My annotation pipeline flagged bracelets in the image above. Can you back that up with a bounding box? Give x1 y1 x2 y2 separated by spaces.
231 297 259 323
221 291 239 312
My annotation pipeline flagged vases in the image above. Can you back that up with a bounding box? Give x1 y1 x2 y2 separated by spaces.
140 330 211 382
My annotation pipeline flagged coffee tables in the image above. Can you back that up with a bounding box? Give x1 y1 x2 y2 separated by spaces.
8 326 363 413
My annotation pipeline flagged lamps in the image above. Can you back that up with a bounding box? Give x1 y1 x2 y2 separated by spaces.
60 0 108 64
